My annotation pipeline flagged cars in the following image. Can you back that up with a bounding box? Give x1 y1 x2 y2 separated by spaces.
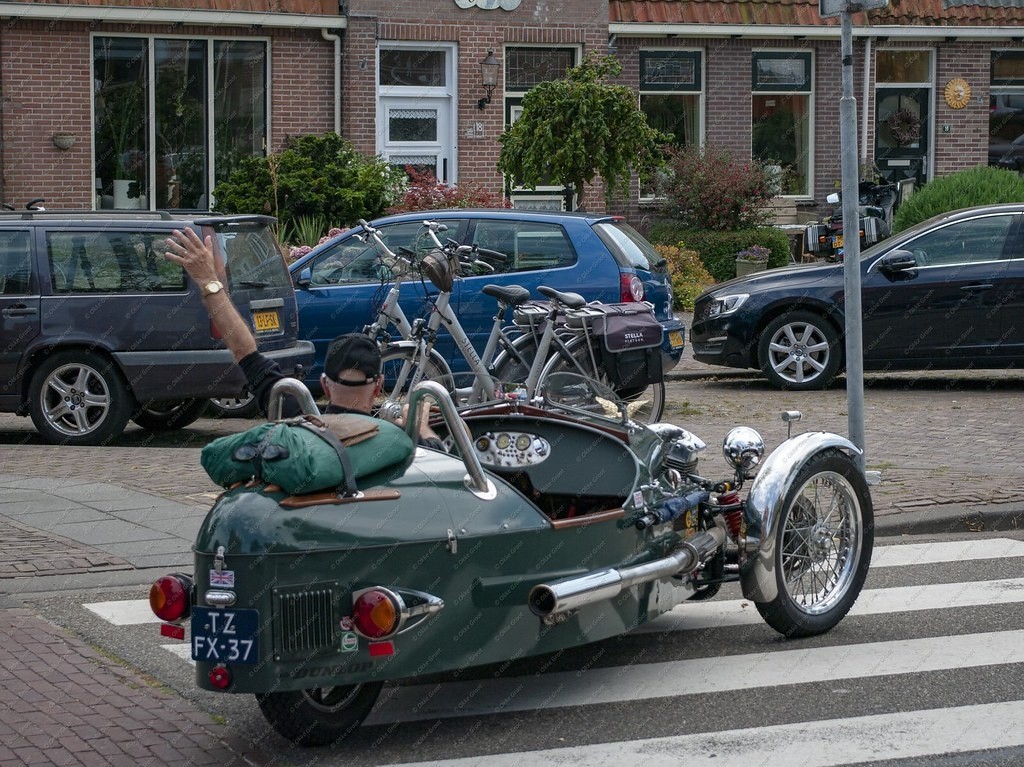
688 202 1024 392
289 209 687 417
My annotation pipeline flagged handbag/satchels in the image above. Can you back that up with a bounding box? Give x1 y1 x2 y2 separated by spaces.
592 312 664 404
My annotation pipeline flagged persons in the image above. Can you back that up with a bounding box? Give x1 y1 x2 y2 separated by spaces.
166 226 449 454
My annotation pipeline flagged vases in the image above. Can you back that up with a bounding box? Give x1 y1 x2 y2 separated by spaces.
736 260 767 277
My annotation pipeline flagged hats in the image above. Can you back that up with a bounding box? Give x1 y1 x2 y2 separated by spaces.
324 332 382 387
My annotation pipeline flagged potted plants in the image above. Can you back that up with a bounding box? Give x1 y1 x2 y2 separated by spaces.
54 131 77 151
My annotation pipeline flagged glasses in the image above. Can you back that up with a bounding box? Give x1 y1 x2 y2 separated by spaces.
232 442 290 463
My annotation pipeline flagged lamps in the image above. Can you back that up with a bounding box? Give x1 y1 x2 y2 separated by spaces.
479 49 501 109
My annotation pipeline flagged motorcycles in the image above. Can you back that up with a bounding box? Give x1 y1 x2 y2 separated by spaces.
150 378 876 748
817 172 917 265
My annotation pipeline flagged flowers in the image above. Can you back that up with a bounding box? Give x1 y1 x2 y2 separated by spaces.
737 244 772 261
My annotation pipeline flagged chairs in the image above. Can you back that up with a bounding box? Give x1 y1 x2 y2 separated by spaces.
801 230 817 263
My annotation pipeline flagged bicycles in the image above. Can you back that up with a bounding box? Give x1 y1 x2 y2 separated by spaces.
353 212 667 429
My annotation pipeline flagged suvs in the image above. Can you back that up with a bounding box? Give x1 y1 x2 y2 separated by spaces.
0 196 318 448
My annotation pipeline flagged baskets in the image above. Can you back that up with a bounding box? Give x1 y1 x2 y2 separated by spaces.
735 259 768 278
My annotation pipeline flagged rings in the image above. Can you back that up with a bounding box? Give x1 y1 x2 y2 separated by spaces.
183 250 188 257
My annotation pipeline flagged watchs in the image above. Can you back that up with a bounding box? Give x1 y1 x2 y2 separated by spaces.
202 281 224 297
